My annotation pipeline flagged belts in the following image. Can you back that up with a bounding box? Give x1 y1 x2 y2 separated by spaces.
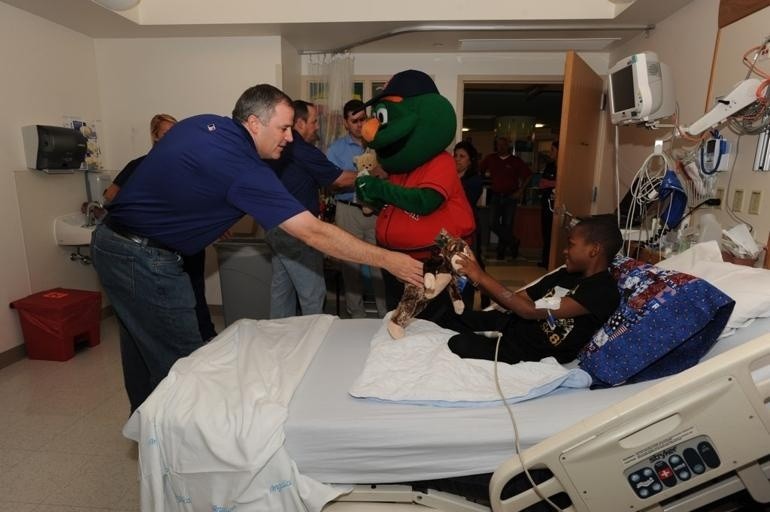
338 200 361 207
102 215 174 251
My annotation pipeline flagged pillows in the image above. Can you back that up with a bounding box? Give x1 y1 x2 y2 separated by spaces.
686 259 770 341
579 258 735 390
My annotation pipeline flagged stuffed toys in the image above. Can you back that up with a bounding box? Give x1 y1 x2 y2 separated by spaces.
357 73 474 340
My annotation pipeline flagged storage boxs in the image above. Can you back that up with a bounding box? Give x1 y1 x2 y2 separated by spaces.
9 287 101 361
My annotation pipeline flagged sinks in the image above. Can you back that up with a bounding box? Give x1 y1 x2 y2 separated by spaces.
52 210 97 246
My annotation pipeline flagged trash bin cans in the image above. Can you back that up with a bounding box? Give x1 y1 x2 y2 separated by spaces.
213 237 296 328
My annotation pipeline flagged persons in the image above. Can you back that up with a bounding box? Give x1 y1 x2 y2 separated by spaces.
89 83 423 416
416 213 625 367
451 132 560 308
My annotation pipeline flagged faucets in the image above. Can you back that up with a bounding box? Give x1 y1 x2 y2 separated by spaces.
86 202 101 217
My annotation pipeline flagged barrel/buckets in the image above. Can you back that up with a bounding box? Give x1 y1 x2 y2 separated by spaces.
212 236 273 330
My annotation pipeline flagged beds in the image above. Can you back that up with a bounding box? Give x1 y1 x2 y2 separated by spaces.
123 244 770 510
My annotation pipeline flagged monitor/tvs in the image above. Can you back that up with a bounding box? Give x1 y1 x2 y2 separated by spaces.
608 54 675 125
